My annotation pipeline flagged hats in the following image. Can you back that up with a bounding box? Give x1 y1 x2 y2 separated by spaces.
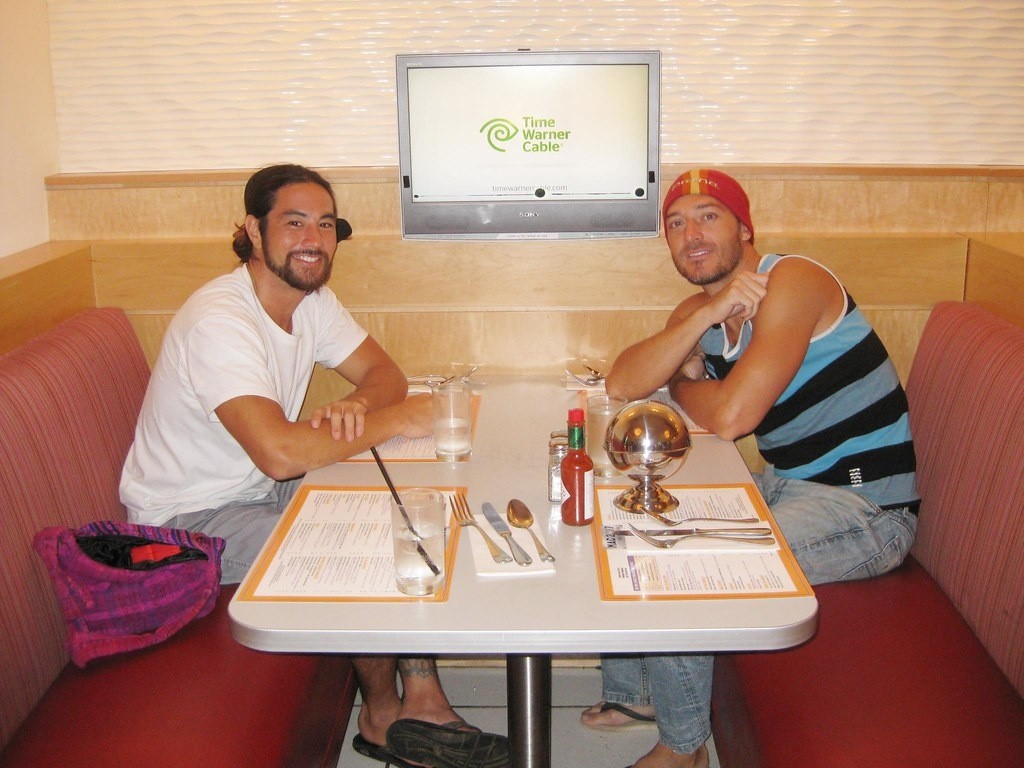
663 170 755 247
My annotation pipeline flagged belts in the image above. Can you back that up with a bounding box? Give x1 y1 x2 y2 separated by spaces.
907 502 920 520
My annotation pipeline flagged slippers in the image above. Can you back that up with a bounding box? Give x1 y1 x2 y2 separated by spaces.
352 732 429 768
580 701 659 731
386 718 510 768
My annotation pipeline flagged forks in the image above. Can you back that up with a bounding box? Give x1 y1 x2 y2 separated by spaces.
450 493 513 564
628 522 776 549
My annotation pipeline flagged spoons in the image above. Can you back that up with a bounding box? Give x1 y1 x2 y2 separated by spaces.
641 507 759 527
507 499 556 564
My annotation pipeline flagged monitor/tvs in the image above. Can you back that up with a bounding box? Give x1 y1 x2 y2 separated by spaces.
395 50 661 239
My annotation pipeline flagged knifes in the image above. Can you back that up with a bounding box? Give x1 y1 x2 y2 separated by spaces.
566 364 593 387
613 528 772 537
482 502 532 567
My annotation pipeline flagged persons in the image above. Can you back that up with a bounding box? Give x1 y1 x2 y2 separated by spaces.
583 168 922 768
119 163 512 768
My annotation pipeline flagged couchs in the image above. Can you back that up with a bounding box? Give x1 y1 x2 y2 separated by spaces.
0 297 357 768
710 301 1024 767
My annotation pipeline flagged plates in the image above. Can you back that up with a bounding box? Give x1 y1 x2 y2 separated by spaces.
405 375 448 396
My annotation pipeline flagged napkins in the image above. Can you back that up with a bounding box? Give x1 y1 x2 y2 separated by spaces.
466 512 557 577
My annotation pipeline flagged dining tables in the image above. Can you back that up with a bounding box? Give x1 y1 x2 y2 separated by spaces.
228 371 819 767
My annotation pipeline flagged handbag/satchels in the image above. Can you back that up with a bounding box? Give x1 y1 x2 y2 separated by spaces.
34 520 226 670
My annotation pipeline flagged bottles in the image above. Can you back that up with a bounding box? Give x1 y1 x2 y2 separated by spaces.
549 429 570 503
560 409 595 528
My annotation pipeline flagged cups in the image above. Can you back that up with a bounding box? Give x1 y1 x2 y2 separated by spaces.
431 382 473 463
586 394 629 478
388 488 445 597
